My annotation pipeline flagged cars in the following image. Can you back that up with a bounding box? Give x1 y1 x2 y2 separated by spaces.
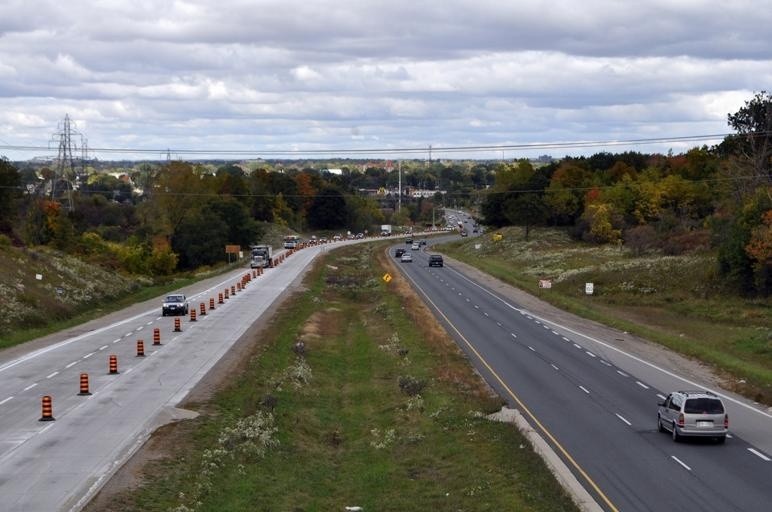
424 208 487 237
309 231 365 244
406 238 426 250
405 230 411 235
402 253 413 262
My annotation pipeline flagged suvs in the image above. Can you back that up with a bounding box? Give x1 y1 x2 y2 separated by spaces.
161 293 189 317
396 248 405 256
657 390 729 445
428 255 444 267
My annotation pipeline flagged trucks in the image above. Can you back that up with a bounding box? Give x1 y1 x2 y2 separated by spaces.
282 235 301 248
380 225 392 235
250 244 272 269
